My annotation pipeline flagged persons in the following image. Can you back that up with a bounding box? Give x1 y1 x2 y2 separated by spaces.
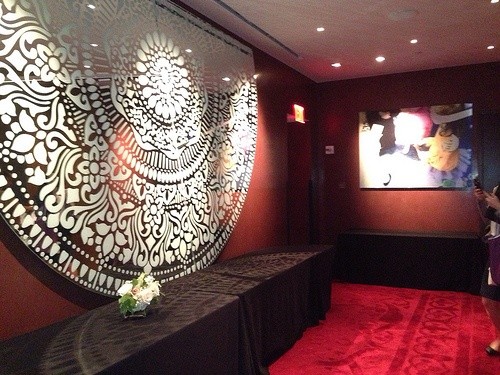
473 177 500 353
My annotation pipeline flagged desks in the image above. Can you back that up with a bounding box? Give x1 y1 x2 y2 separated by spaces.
0 231 486 375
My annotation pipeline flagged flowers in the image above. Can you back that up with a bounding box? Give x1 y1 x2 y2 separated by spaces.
119 272 161 318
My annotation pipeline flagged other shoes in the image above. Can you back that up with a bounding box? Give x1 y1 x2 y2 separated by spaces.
486 345 499 354
